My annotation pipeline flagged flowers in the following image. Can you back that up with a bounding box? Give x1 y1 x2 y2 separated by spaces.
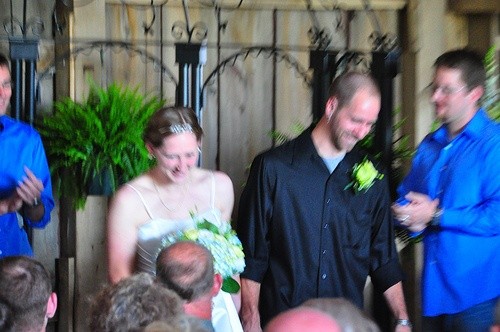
154 208 246 294
341 154 384 195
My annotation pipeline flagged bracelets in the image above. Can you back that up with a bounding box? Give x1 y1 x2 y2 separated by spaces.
395 319 410 326
428 205 442 228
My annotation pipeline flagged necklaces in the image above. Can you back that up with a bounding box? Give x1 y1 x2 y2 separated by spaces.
153 177 186 212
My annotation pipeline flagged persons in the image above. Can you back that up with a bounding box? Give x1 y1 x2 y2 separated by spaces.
85 105 246 332
236 47 500 332
0 51 58 332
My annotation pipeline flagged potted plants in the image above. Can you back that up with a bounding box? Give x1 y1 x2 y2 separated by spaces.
41 68 166 210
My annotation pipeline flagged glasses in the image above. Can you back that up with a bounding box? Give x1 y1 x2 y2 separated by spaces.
431 82 469 95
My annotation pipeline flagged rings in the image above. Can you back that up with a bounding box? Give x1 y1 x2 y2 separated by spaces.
402 215 409 220
